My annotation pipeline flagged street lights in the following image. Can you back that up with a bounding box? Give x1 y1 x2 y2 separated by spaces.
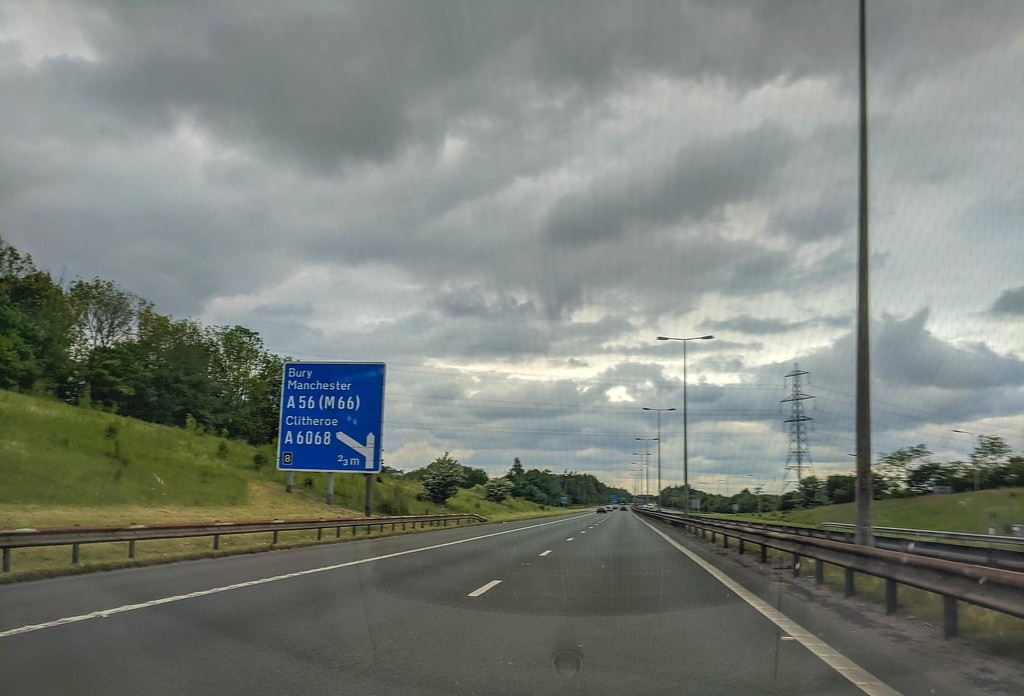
628 437 659 511
643 407 675 512
656 334 716 518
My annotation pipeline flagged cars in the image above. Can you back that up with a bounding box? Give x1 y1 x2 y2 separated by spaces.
620 506 627 511
612 506 618 510
596 506 608 514
604 505 613 512
648 504 654 509
